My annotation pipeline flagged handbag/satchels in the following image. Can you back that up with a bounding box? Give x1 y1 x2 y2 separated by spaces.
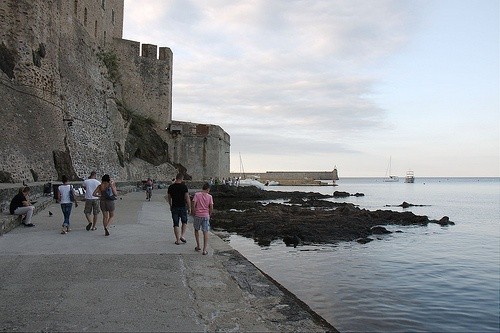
104 181 117 201
70 185 76 202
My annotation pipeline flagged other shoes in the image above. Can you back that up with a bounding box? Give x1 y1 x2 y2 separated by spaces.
180 238 187 243
146 198 148 199
61 228 67 233
105 227 110 236
22 222 35 227
195 247 201 250
175 240 179 245
203 251 208 255
86 222 92 231
67 228 71 232
91 227 97 230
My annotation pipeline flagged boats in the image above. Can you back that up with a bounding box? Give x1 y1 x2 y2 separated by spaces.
239 151 260 182
405 169 416 183
268 180 280 185
378 156 399 182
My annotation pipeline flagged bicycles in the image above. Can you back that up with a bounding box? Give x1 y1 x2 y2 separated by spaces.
146 187 154 202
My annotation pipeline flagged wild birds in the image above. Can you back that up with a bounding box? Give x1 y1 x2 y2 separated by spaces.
48 211 53 216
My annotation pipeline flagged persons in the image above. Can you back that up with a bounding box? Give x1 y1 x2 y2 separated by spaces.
10 187 35 227
145 178 154 199
191 182 214 255
92 174 118 235
209 176 240 187
58 174 80 234
167 173 191 244
81 171 101 231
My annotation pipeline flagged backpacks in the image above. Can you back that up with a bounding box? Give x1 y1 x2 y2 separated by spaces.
147 180 152 187
